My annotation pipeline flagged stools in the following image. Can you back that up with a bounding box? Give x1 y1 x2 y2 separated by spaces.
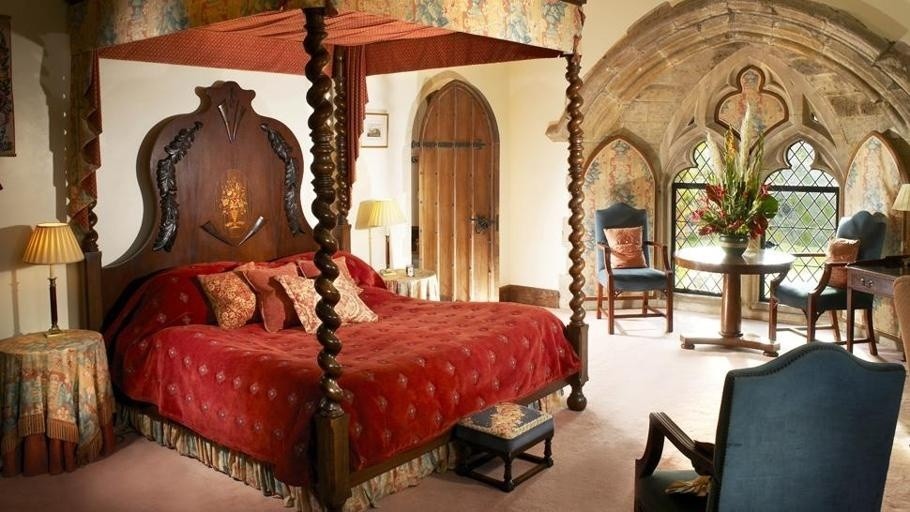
452 404 554 491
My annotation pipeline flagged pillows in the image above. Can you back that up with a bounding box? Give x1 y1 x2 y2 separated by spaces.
194 262 264 327
601 225 649 270
296 255 366 299
273 272 381 335
825 233 860 290
240 262 305 335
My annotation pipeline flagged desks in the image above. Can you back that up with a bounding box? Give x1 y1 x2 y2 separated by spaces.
377 268 442 301
671 242 796 358
846 255 910 360
1 329 118 479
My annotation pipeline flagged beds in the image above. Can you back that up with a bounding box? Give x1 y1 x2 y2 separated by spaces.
63 248 590 511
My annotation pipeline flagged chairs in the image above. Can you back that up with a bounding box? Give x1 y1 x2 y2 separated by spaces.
634 341 906 512
892 275 909 373
769 211 889 357
594 203 673 335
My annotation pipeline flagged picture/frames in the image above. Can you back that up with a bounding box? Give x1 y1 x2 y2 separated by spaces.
358 112 389 149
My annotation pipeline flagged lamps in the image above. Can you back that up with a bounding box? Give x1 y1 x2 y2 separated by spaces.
23 221 86 336
383 200 408 276
891 184 910 267
353 199 383 279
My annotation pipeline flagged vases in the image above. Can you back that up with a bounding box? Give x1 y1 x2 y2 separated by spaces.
718 228 749 256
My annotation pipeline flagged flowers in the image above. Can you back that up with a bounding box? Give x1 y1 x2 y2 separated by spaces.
689 103 781 237
